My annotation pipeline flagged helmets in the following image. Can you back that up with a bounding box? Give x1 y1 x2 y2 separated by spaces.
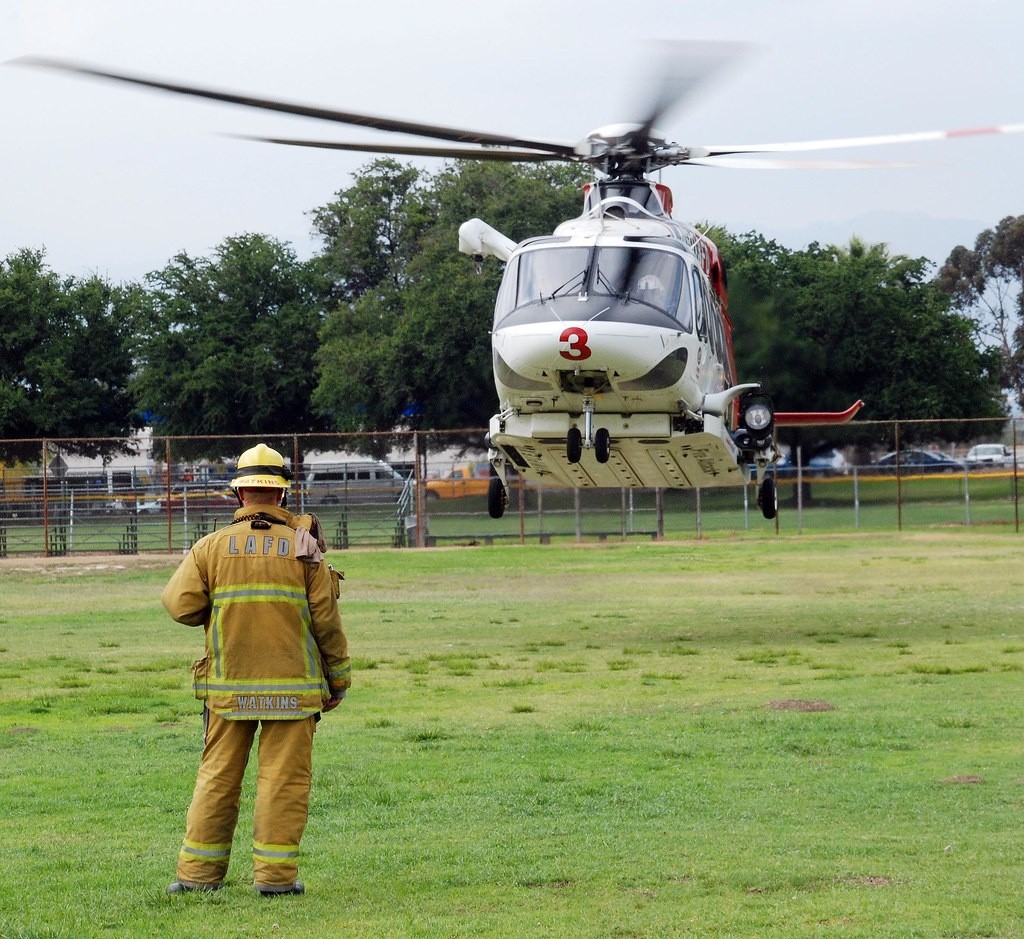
230 444 294 508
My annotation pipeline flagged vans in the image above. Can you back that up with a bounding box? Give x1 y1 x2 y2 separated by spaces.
306 460 409 507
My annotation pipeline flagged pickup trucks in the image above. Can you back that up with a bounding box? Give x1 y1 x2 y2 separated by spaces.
413 466 491 502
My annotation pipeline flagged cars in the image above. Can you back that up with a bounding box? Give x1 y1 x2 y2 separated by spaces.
964 442 1014 470
749 442 849 478
873 449 964 476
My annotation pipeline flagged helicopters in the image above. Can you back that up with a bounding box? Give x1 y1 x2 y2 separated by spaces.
6 39 1024 518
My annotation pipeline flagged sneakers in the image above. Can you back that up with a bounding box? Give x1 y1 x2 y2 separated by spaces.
260 881 305 898
168 883 222 894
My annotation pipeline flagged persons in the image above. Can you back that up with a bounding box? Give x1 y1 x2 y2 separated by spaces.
160 444 350 899
